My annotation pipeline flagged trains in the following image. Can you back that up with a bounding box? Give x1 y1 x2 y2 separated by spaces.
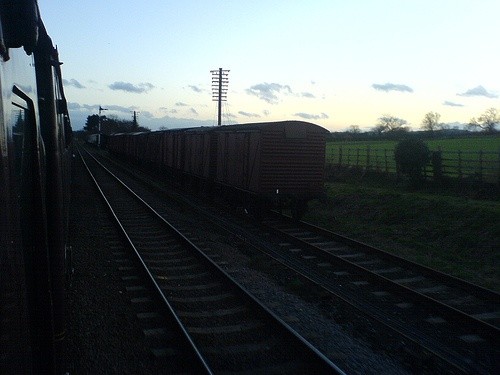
76 121 330 219
